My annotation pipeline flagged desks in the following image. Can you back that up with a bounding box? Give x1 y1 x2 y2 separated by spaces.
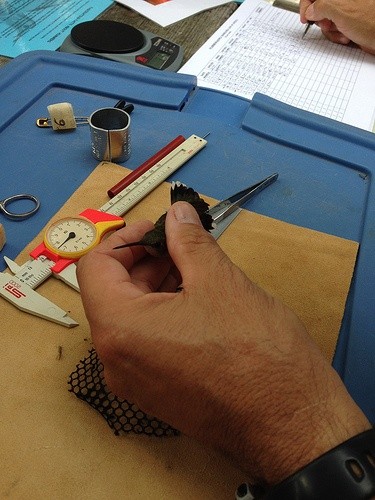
0 0 299 69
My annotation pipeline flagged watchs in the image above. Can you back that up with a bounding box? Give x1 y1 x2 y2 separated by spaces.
236 427 375 500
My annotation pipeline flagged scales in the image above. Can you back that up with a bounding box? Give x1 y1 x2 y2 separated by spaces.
60 18 185 77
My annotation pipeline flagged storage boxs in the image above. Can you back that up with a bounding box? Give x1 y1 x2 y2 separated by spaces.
0 49 375 428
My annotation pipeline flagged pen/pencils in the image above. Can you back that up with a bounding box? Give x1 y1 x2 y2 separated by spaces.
299 0 326 40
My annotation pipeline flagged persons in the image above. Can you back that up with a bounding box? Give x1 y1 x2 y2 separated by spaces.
75 0 375 500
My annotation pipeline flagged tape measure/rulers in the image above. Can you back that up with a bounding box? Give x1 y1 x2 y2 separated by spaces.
161 200 243 287
0 134 208 328
